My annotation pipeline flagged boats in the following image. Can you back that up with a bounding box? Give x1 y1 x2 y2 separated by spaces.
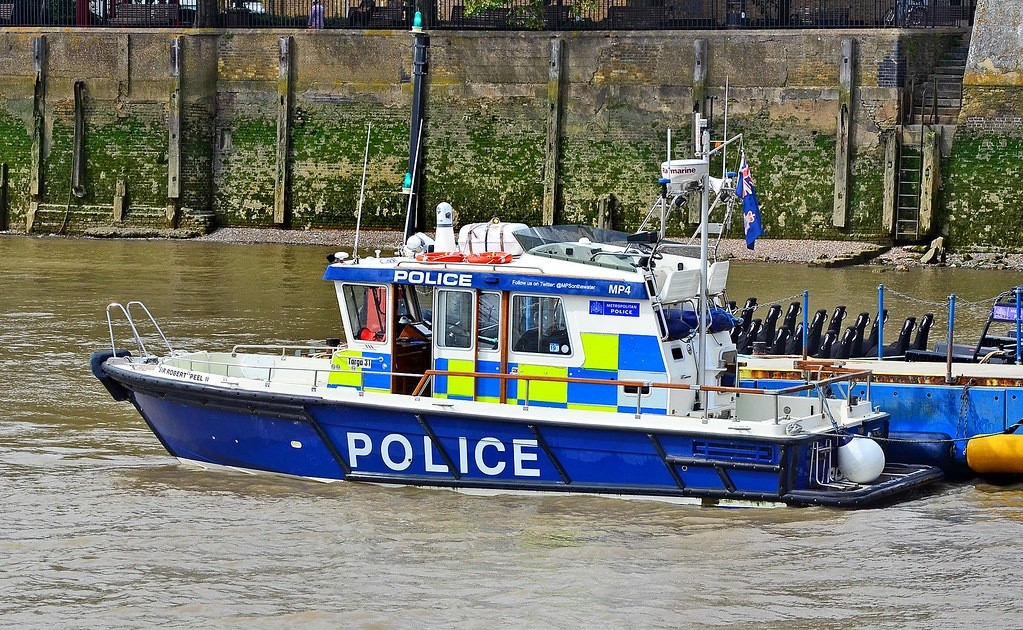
88 116 948 512
710 263 1023 483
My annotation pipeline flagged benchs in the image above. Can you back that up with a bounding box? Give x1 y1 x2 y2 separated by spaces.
0 3 15 25
348 6 422 26
902 4 971 27
451 5 571 29
795 7 852 26
107 3 181 25
605 6 674 29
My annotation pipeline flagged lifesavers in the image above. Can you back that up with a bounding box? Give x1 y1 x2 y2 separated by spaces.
468 252 512 264
416 252 464 265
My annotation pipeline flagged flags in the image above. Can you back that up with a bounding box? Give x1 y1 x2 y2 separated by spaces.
734 153 763 250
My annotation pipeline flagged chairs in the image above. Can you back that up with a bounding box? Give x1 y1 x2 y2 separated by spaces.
724 298 934 361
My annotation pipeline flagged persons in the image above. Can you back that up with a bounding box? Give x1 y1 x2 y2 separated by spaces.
308 0 324 29
